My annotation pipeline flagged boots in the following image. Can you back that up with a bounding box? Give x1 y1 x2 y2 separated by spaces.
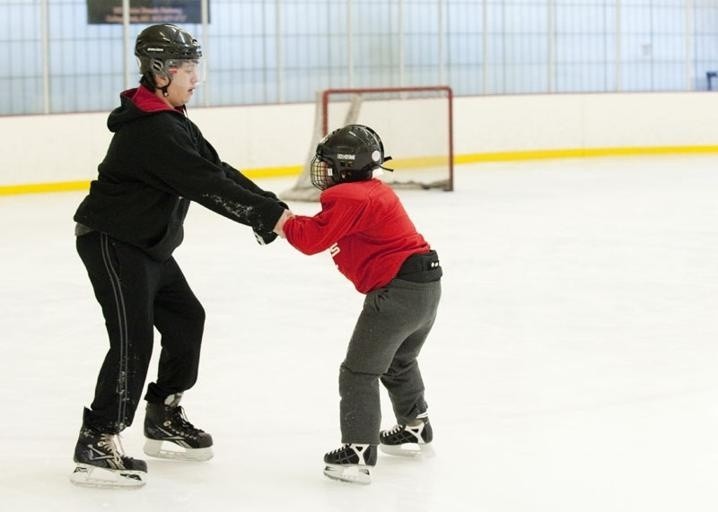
143 391 215 450
71 408 150 472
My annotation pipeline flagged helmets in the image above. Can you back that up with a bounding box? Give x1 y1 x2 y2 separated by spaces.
133 22 204 76
309 123 394 191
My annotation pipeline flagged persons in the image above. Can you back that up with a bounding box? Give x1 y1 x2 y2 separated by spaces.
71 25 292 472
284 125 442 467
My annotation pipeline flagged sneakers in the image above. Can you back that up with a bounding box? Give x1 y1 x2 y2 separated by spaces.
323 442 379 468
379 408 435 446
